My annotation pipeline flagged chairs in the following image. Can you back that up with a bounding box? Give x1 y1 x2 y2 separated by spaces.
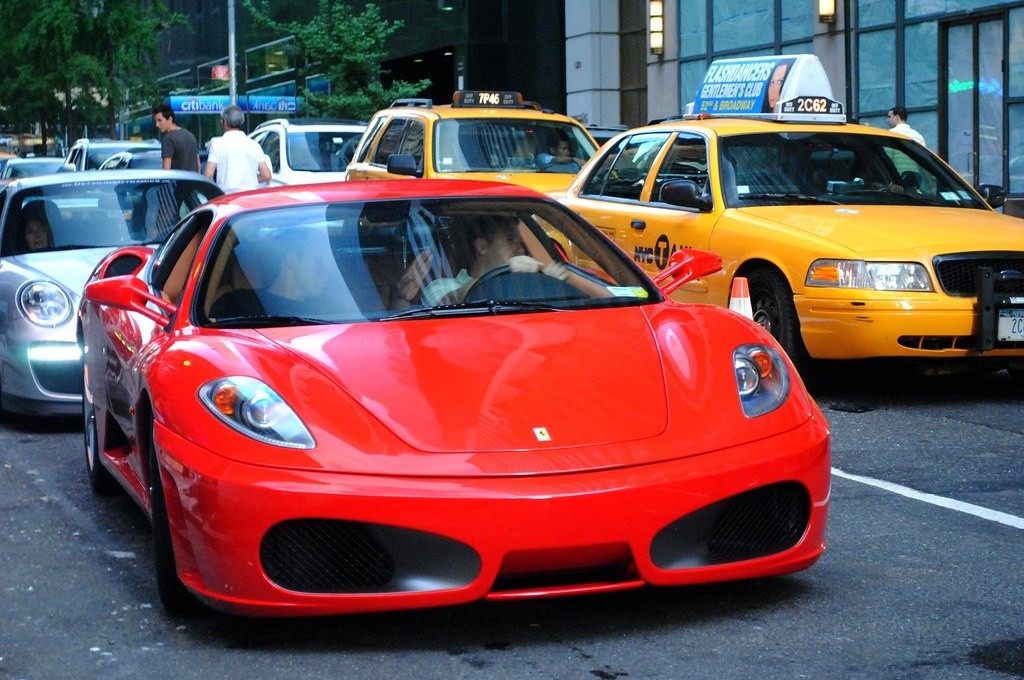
212 249 264 304
20 200 67 245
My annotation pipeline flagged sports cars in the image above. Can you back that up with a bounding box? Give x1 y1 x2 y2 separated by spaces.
73 176 833 626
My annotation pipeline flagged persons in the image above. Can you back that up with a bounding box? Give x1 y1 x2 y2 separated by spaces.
203 104 274 195
534 128 639 178
206 226 436 321
16 213 51 250
418 215 615 310
148 103 201 245
848 106 938 197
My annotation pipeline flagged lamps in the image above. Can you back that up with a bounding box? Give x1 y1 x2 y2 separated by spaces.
650 0 664 54
818 0 835 23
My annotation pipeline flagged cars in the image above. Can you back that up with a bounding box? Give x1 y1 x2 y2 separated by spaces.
531 53 1024 403
0 91 628 416
